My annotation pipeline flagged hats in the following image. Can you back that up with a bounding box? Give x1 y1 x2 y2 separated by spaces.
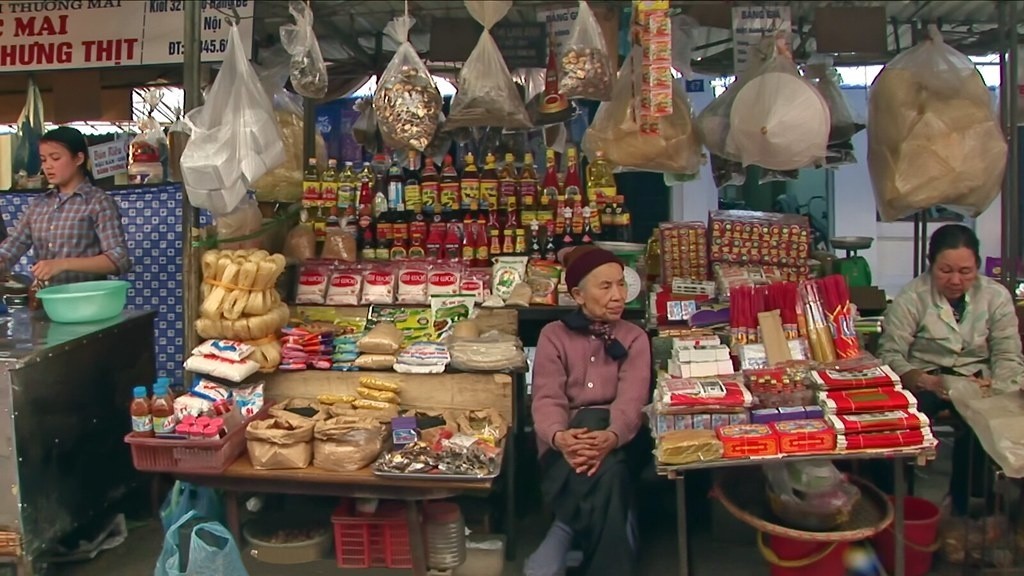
558 246 624 299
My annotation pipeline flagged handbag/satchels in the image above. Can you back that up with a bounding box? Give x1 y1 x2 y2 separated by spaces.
691 30 865 185
178 21 287 215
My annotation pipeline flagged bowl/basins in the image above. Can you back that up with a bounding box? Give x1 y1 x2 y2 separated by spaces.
241 511 334 563
34 281 129 323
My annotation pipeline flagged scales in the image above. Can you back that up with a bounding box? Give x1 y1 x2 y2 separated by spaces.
830 235 875 289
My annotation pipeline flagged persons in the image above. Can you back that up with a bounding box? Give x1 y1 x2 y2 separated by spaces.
874 224 1024 519
0 126 132 289
523 245 651 576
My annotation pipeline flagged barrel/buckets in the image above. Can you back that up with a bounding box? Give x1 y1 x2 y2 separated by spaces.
874 496 943 575
755 528 851 576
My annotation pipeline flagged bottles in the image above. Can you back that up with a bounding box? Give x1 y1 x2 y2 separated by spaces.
130 377 226 442
423 501 466 570
299 150 632 268
128 153 163 185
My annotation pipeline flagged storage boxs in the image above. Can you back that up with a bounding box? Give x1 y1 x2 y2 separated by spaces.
331 497 412 569
125 398 276 474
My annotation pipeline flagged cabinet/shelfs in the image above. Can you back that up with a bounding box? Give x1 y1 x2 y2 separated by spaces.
172 454 507 576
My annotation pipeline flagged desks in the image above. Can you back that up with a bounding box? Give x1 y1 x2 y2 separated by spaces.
657 447 936 576
